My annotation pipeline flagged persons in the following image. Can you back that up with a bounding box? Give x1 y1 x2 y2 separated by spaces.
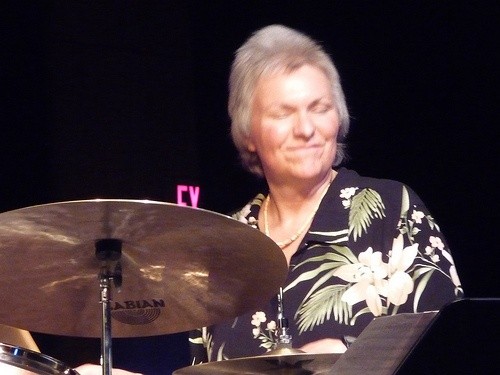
72 25 464 375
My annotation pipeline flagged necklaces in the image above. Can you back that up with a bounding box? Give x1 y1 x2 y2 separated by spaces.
262 174 334 248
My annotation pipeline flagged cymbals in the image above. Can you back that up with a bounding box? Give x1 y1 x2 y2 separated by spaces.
0 196 290 338
172 326 344 375
